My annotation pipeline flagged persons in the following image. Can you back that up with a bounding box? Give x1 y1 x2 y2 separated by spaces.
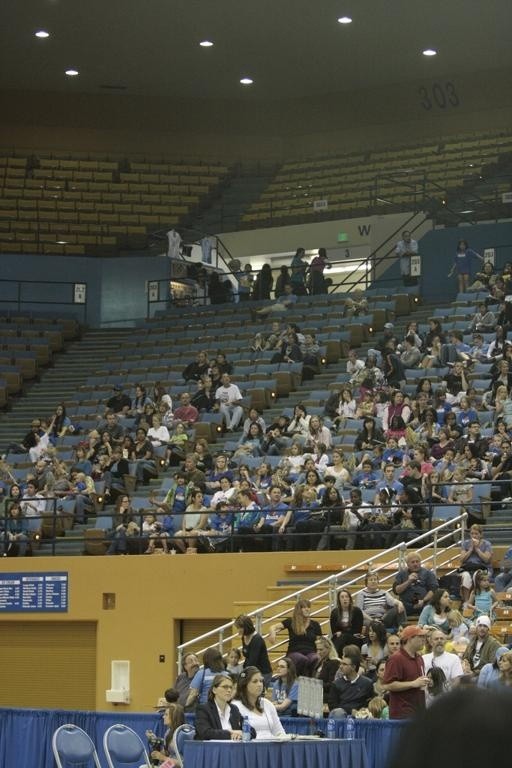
230 404 352 553
316 319 512 552
0 349 243 555
450 241 511 334
177 247 332 308
145 525 510 768
394 231 420 287
248 321 319 380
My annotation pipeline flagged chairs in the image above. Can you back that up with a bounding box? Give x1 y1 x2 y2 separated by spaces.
53 722 198 768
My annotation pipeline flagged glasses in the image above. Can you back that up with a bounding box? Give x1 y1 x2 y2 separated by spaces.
217 685 233 690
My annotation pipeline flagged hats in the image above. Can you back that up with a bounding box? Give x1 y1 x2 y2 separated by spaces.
384 323 394 330
31 419 41 427
400 626 427 641
113 384 123 391
475 616 490 630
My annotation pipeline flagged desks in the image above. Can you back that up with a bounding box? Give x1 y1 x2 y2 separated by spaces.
184 738 367 767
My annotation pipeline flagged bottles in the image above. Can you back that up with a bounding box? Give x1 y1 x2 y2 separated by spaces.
327 716 336 739
345 714 355 739
242 715 251 741
